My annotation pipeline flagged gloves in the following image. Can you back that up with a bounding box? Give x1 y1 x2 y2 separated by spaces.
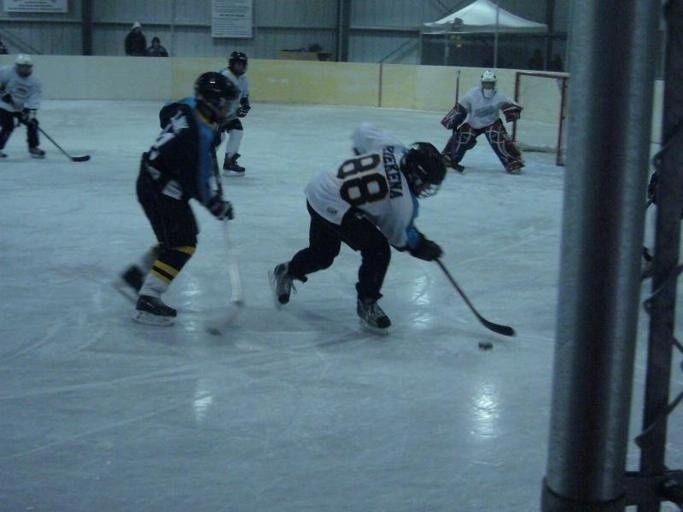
20 109 37 129
209 198 234 221
402 229 441 260
1 90 11 102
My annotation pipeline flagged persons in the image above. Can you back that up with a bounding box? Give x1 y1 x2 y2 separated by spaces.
125 22 147 56
0 56 44 157
442 71 524 172
274 125 446 329
123 72 241 316
147 37 167 57
219 53 251 172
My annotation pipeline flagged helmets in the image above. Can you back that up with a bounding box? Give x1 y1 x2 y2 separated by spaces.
229 52 247 75
194 71 239 124
481 71 497 100
15 53 32 77
399 141 447 200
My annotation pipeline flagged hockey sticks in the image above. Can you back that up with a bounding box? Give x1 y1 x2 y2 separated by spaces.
453 75 467 175
209 150 243 334
435 258 516 337
19 115 91 163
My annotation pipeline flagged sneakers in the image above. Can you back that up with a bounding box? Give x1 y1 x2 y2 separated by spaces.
135 297 176 318
357 283 392 328
222 152 245 171
123 265 144 291
272 260 309 304
28 147 44 155
